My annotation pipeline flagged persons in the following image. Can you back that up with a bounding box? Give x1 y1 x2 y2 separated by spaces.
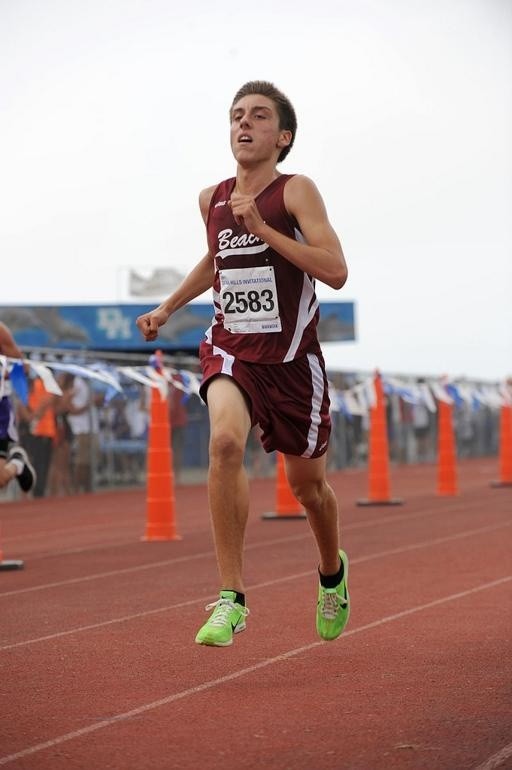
0 319 188 500
328 378 431 466
134 80 352 647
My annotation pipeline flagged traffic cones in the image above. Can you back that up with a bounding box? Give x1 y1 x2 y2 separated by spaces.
260 452 307 521
490 405 512 487
356 369 402 506
434 401 459 497
140 350 181 544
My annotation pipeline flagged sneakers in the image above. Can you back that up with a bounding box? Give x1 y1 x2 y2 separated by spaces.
316 550 349 639
196 591 247 646
11 446 37 493
